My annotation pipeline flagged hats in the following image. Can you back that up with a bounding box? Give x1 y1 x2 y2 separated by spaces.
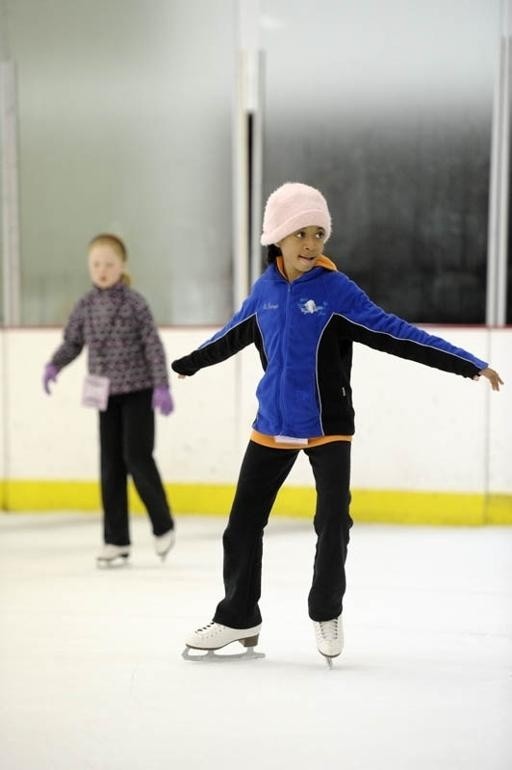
260 181 332 248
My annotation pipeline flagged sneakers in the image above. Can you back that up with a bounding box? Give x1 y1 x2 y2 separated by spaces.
315 614 344 658
185 616 263 651
154 528 176 554
97 541 131 562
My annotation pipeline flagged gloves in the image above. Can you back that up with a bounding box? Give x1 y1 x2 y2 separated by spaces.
151 384 175 418
41 365 59 393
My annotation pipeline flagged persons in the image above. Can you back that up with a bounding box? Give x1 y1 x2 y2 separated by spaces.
172 183 504 658
43 235 169 558
